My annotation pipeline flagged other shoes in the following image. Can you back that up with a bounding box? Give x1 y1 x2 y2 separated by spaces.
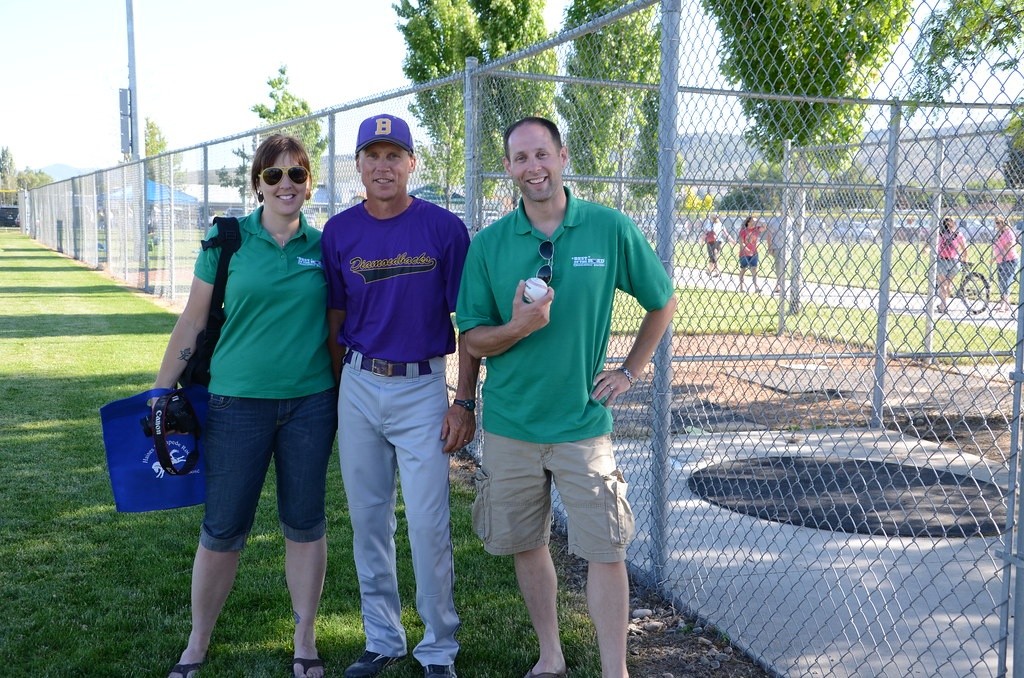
740 287 763 293
936 305 948 313
705 271 720 278
994 305 1011 313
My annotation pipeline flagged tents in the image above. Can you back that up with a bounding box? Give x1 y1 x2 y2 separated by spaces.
95 178 201 228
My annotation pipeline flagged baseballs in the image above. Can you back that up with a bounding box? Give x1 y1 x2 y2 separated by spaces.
523 278 548 303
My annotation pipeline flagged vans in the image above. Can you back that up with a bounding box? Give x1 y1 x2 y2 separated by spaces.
222 207 244 218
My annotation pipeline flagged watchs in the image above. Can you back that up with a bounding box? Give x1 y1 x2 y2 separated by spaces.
454 399 476 410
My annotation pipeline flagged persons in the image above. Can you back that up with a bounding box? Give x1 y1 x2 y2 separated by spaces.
151 135 338 678
320 115 481 678
990 215 1020 312
703 213 735 276
766 209 795 294
455 117 677 678
738 216 767 293
935 217 969 313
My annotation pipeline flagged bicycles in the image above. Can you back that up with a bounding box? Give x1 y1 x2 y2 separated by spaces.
925 252 991 315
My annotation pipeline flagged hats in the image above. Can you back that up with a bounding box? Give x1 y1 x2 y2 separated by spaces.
354 114 415 154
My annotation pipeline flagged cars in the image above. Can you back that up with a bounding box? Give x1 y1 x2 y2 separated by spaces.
632 217 1022 242
448 209 501 222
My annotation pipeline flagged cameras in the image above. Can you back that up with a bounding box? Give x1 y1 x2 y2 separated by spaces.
140 394 198 437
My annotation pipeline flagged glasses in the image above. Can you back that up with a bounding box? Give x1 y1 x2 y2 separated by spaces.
535 238 554 286
258 166 311 186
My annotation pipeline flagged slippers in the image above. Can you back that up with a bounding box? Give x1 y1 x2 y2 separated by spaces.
518 663 574 678
292 649 326 678
168 644 209 678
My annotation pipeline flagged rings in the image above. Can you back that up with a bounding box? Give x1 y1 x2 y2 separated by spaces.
464 440 468 443
609 385 613 391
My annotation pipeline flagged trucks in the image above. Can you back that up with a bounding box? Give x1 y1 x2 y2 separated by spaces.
196 206 218 228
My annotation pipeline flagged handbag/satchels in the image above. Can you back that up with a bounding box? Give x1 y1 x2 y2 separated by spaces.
99 387 208 512
178 307 228 389
706 230 716 245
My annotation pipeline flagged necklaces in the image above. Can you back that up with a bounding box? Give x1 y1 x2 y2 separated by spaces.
276 237 289 246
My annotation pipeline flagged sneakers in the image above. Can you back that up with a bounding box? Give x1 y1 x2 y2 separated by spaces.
423 663 458 678
343 650 408 678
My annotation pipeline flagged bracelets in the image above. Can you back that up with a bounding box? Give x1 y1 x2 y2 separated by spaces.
619 366 634 387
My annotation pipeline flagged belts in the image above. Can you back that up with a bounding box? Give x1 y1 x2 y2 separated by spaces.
344 348 432 376
